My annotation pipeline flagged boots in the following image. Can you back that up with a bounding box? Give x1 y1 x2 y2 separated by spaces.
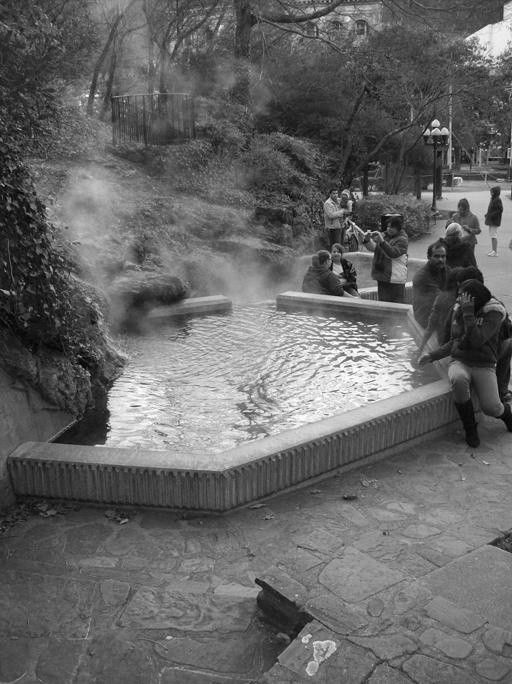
452 399 480 447
496 403 512 432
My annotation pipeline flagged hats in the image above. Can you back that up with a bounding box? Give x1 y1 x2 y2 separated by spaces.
446 223 462 236
342 189 350 198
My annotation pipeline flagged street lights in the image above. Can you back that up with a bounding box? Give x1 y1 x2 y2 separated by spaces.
422 119 451 212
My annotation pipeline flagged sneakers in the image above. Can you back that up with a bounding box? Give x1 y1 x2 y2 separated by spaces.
488 251 498 256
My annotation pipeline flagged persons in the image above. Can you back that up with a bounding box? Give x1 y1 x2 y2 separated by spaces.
413 186 512 448
302 250 344 298
362 216 408 303
323 188 352 253
338 188 353 229
328 243 362 300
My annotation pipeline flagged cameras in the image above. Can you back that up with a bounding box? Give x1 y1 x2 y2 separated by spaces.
367 232 377 238
